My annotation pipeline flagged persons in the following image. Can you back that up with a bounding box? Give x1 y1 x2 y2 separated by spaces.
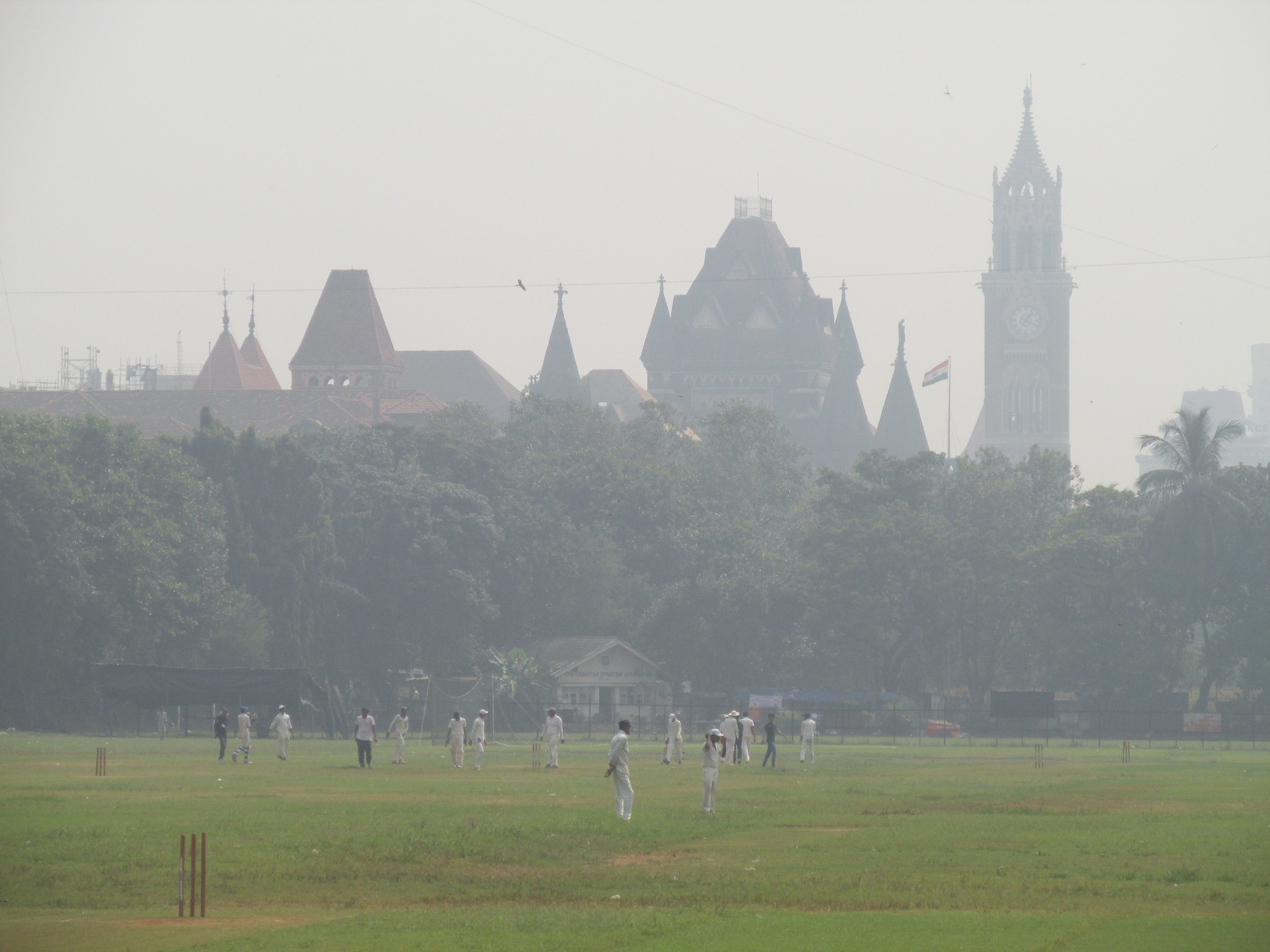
663 714 683 764
445 711 467 768
702 729 727 815
540 708 565 768
214 707 228 760
269 705 293 760
469 710 488 771
232 707 252 764
155 706 166 740
354 707 377 768
800 712 816 764
605 719 634 822
386 707 409 764
762 713 787 767
720 710 743 765
739 711 756 764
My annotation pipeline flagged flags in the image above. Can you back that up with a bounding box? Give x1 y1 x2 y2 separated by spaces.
922 359 948 387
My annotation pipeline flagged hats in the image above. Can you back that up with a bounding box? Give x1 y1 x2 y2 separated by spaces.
668 713 675 721
240 707 248 712
401 707 408 711
479 710 488 715
545 707 556 712
278 705 285 710
728 710 739 716
802 713 810 717
618 719 631 729
710 729 724 737
723 715 729 718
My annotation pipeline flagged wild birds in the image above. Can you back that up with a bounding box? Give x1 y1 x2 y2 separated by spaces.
516 279 526 291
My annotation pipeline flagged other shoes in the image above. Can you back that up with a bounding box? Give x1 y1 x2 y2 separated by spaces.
677 760 683 764
545 764 558 768
231 752 237 762
367 762 372 768
737 759 741 764
242 760 251 764
393 760 404 764
277 755 286 760
709 810 715 814
663 759 669 765
475 768 480 771
453 761 461 768
800 760 804 763
745 761 749 764
